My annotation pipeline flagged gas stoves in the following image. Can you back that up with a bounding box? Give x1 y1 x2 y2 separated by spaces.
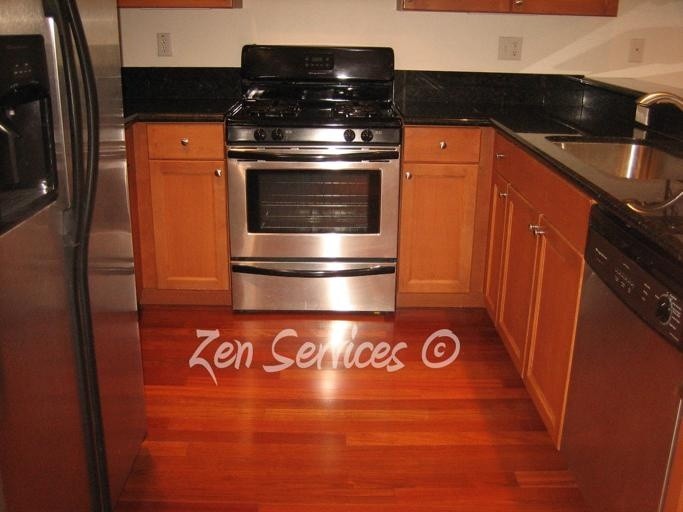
226 79 402 146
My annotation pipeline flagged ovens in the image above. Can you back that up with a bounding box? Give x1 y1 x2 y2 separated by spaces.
227 146 400 312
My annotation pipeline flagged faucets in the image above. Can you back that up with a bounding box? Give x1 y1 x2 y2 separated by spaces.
625 192 683 216
634 92 683 139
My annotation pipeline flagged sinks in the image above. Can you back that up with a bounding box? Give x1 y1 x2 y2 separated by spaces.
545 135 683 182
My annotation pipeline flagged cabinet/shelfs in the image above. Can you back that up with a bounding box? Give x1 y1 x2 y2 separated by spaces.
395 124 482 296
480 131 595 453
133 124 233 292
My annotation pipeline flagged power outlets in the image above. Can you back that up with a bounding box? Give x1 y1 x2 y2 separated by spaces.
497 35 523 60
157 32 173 57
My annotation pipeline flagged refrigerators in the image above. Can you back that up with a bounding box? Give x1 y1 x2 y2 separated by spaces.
2 1 150 512
